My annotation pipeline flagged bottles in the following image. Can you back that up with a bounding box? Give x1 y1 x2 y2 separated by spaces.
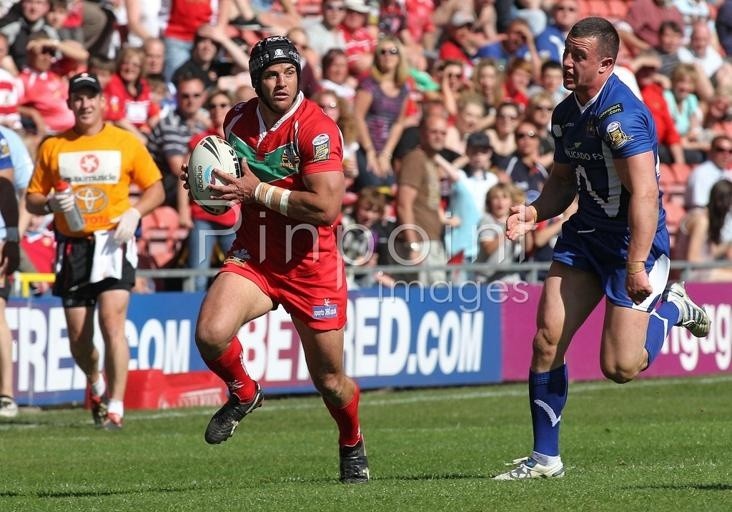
55 181 86 232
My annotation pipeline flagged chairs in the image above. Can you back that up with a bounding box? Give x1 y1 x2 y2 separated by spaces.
574 1 732 261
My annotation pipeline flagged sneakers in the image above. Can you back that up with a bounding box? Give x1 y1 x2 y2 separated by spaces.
1 395 17 417
205 382 264 445
662 282 710 338
495 452 565 480
338 433 369 486
90 390 123 432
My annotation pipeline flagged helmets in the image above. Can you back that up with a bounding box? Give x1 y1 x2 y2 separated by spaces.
249 37 300 85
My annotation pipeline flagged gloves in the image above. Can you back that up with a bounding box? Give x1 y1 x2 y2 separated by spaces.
113 208 141 246
50 195 74 213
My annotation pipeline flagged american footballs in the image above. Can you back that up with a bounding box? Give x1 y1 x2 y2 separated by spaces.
189 135 241 216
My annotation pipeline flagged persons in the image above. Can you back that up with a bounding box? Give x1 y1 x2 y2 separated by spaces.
494 16 711 481
177 34 371 487
0 125 33 418
0 0 731 298
23 71 167 430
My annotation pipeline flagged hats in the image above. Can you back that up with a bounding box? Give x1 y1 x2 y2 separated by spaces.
70 73 99 91
